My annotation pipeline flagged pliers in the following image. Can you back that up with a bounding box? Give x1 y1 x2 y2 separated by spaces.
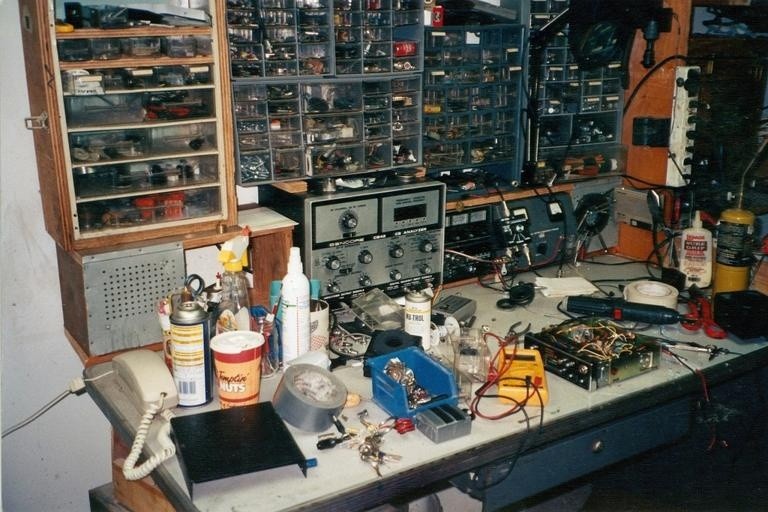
680 283 728 339
504 321 531 342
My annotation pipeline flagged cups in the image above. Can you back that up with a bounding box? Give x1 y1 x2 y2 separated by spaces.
209 331 266 408
308 299 328 351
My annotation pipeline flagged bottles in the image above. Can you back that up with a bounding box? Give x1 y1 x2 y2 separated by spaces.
403 288 431 353
170 301 214 411
280 246 312 374
250 316 279 377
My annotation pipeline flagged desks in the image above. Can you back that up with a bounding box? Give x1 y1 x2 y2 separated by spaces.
84 250 768 511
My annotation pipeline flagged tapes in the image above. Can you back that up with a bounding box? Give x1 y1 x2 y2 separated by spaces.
272 363 348 432
373 329 414 356
622 279 679 311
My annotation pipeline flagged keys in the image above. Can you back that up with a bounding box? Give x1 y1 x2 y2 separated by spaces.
314 423 402 478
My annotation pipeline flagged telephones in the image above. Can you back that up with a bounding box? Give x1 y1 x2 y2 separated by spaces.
111 348 179 418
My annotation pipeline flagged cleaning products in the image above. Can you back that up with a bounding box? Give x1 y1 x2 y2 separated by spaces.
220 224 255 333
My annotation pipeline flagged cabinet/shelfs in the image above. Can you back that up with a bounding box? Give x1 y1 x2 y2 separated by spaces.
228 0 625 187
55 203 299 366
17 0 239 251
620 0 768 269
